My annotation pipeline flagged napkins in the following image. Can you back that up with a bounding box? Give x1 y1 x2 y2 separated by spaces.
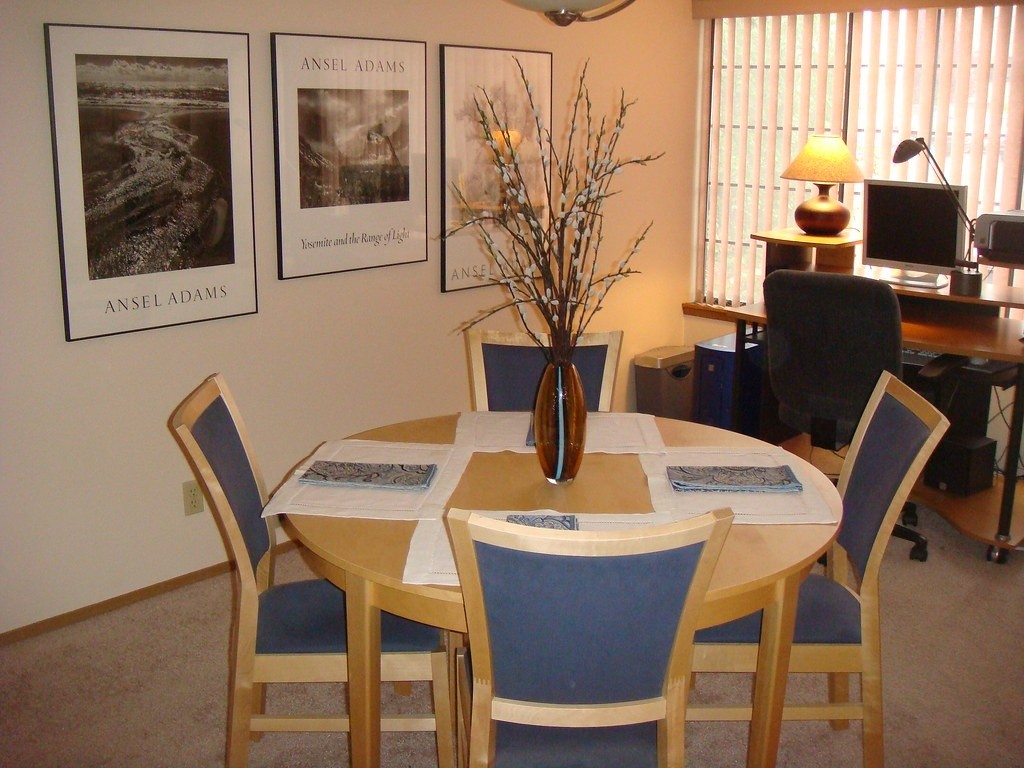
526 411 537 446
506 515 579 531
666 465 804 492
298 459 438 492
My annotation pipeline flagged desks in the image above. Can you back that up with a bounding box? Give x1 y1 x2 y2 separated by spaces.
723 280 1024 564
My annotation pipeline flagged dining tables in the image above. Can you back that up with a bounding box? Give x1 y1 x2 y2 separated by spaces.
260 410 844 768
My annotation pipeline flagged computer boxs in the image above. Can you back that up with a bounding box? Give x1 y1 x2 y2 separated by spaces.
905 364 991 441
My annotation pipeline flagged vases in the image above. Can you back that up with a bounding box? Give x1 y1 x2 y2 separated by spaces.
535 333 587 484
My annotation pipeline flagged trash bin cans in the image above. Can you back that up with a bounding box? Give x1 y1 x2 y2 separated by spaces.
632 345 696 423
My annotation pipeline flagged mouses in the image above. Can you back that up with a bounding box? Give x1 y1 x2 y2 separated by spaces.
970 357 989 365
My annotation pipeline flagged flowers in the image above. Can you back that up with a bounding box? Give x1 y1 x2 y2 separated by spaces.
444 53 667 363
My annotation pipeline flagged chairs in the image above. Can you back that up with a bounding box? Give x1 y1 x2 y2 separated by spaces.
763 270 929 564
464 322 623 413
684 371 951 768
170 373 455 768
444 507 732 768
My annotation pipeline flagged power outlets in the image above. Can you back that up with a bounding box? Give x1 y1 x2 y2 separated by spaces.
182 480 205 517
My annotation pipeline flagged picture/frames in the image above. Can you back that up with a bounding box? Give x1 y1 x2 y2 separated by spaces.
440 45 551 294
270 32 429 281
43 22 258 342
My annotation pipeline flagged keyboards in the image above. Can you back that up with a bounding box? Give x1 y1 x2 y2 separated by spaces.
901 348 941 366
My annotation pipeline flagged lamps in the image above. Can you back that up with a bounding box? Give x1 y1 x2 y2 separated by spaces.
892 136 982 296
781 135 866 235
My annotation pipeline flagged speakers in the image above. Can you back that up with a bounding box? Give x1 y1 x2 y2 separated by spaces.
923 435 998 496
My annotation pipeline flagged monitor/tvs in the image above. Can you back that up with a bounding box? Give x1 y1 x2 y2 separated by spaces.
862 179 967 288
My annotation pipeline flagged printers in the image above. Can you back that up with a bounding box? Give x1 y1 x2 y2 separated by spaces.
972 209 1024 266
